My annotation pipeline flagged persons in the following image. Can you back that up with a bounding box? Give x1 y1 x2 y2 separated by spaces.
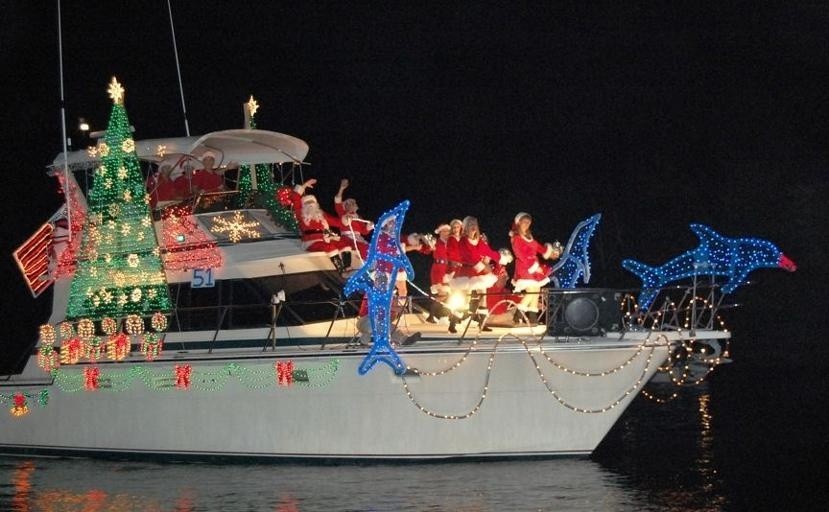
291 176 375 274
405 216 513 334
509 212 559 325
146 152 230 220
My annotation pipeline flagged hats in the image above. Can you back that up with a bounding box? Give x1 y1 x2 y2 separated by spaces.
434 215 472 235
507 210 532 238
158 150 216 173
300 192 356 211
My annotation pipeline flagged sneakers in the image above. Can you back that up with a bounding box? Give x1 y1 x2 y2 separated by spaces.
401 332 421 346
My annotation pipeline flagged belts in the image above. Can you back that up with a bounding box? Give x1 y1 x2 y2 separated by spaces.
303 227 331 236
432 258 475 271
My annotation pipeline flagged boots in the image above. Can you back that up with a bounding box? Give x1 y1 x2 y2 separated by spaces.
512 304 544 328
327 246 361 278
425 307 493 333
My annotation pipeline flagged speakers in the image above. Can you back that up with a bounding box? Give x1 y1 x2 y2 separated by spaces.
547 288 615 337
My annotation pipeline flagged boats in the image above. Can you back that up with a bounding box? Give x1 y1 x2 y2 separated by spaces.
1 3 741 464
0 446 639 511
